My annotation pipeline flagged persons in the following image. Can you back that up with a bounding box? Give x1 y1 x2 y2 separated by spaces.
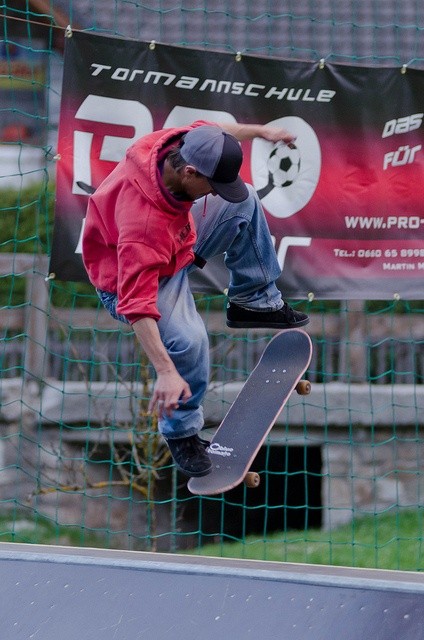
81 119 309 478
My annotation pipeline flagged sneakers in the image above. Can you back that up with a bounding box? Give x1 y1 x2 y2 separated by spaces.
165 430 214 477
225 301 309 330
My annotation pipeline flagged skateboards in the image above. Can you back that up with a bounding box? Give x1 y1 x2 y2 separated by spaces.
185 327 314 497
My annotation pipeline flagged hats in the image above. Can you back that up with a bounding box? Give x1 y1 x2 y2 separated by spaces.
180 122 249 206
